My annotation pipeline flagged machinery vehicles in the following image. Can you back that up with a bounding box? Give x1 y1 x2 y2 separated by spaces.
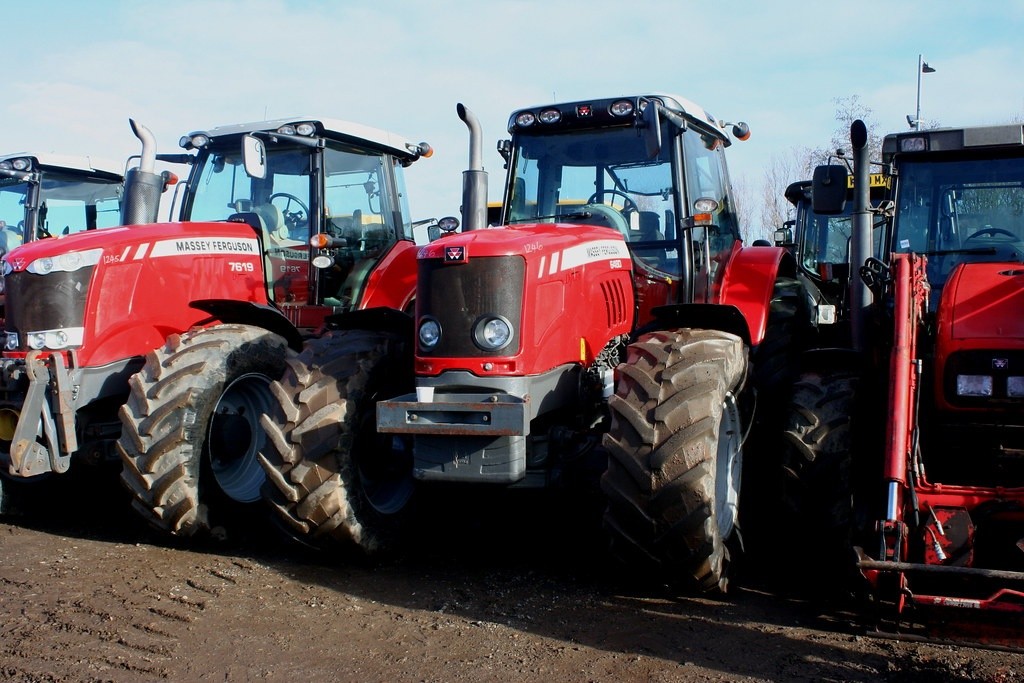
254 91 834 627
1 151 137 264
0 116 437 544
774 149 965 358
769 115 1024 652
